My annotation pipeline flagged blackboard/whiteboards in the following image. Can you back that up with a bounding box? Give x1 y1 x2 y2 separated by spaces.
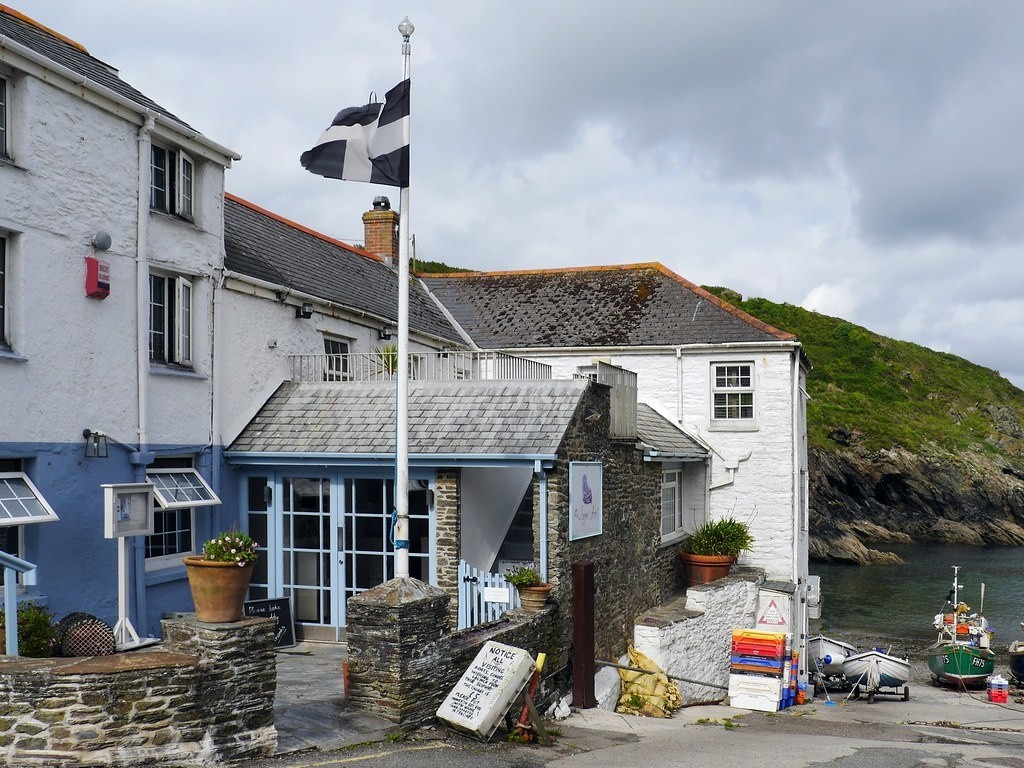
243 596 296 650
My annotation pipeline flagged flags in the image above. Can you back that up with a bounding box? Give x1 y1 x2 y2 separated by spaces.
299 77 411 187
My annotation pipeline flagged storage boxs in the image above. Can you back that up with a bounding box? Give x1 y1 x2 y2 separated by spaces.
985 627 995 640
944 615 954 626
728 629 808 713
987 680 1008 703
957 624 969 634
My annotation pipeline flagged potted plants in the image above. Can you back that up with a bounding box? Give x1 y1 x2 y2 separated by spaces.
677 498 757 588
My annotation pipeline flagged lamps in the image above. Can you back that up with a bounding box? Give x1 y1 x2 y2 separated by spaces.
83 429 108 458
296 302 314 318
439 345 451 359
264 486 271 502
377 325 392 341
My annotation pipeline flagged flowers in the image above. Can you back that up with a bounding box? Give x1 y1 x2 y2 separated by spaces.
200 521 261 567
503 565 542 587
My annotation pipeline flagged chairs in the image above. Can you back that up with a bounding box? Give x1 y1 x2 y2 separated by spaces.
55 611 115 657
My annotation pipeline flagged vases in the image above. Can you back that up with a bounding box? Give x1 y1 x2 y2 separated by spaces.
184 555 256 623
518 581 552 611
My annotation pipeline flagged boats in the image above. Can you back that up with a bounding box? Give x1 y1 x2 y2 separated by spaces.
926 564 995 687
1009 641 1024 683
842 650 911 688
807 633 859 673
